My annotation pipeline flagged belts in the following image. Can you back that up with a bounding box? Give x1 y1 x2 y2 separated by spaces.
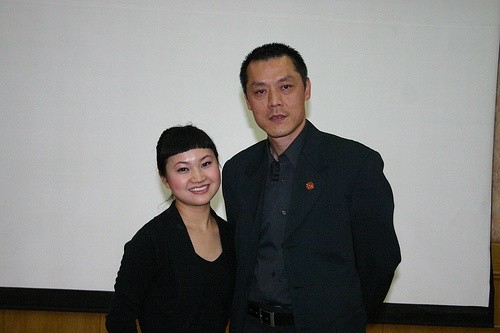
248 305 296 329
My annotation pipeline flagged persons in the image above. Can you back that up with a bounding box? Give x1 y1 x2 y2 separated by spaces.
105 125 234 333
223 43 402 333
